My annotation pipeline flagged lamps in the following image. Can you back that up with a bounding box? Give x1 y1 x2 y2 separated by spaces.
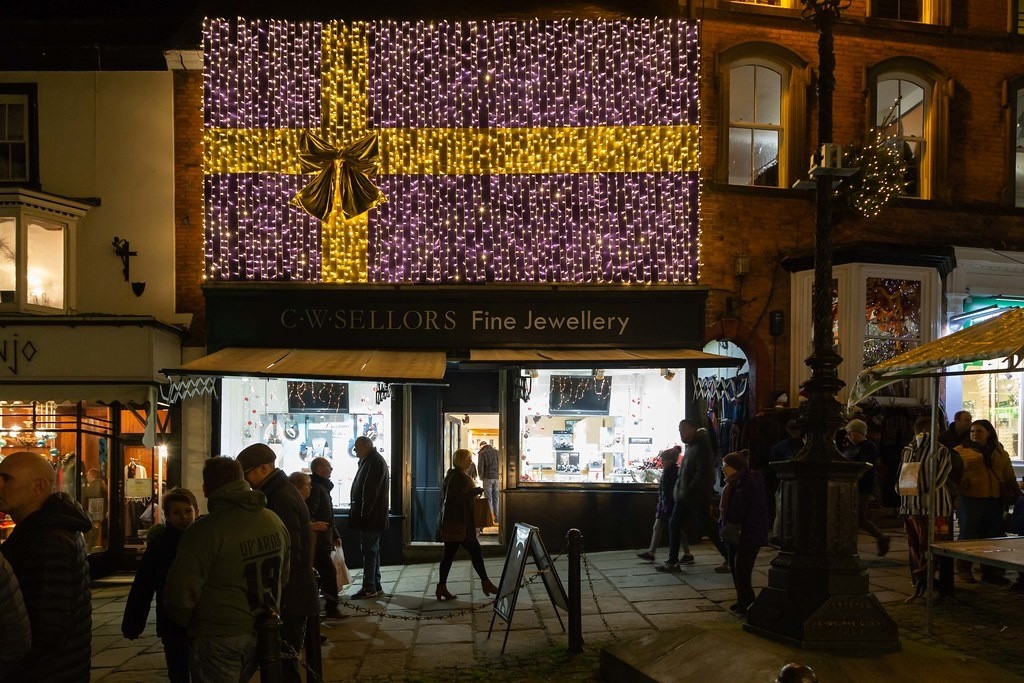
661 368 675 382
592 368 605 380
525 368 539 378
35 401 57 439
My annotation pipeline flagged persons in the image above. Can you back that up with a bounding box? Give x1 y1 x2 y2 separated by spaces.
82 468 107 549
721 448 771 613
637 445 695 566
120 443 350 682
841 419 891 557
54 452 86 514
478 440 500 523
0 451 93 683
654 419 730 573
345 435 391 602
435 449 498 600
895 409 1024 597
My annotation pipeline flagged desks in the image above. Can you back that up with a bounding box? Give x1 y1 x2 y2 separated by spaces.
928 535 1024 598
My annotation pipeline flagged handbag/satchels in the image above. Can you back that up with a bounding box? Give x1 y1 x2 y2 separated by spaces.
331 546 351 592
440 520 466 542
999 481 1013 502
720 522 742 545
474 488 495 527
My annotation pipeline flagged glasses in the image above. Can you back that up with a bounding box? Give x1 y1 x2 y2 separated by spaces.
243 467 258 476
721 464 729 469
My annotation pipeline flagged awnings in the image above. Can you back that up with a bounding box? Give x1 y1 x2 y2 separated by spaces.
158 345 450 395
460 345 747 378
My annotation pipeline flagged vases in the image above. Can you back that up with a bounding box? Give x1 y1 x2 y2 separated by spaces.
644 469 666 483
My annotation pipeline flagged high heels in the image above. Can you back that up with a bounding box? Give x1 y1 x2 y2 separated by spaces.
436 583 457 600
482 580 498 596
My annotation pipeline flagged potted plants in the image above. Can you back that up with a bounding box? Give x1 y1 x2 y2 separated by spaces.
267 433 284 468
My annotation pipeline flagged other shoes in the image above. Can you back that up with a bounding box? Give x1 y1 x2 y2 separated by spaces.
730 602 747 612
878 533 890 556
958 572 974 583
981 573 1010 585
637 552 655 561
911 576 938 588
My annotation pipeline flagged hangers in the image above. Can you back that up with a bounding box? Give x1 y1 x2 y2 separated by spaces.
125 458 146 471
125 497 147 507
152 474 167 486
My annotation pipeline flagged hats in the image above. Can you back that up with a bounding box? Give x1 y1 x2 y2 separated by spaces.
453 449 472 468
234 443 276 467
845 419 867 436
723 449 751 472
660 446 681 464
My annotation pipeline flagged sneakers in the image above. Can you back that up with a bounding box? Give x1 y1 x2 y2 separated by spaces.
654 562 681 572
715 561 730 573
326 612 351 620
351 587 378 599
375 584 384 594
680 554 695 564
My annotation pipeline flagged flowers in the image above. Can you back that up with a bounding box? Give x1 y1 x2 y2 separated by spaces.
638 455 683 470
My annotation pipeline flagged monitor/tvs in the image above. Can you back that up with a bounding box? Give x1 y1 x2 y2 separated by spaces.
549 375 612 415
288 381 348 414
